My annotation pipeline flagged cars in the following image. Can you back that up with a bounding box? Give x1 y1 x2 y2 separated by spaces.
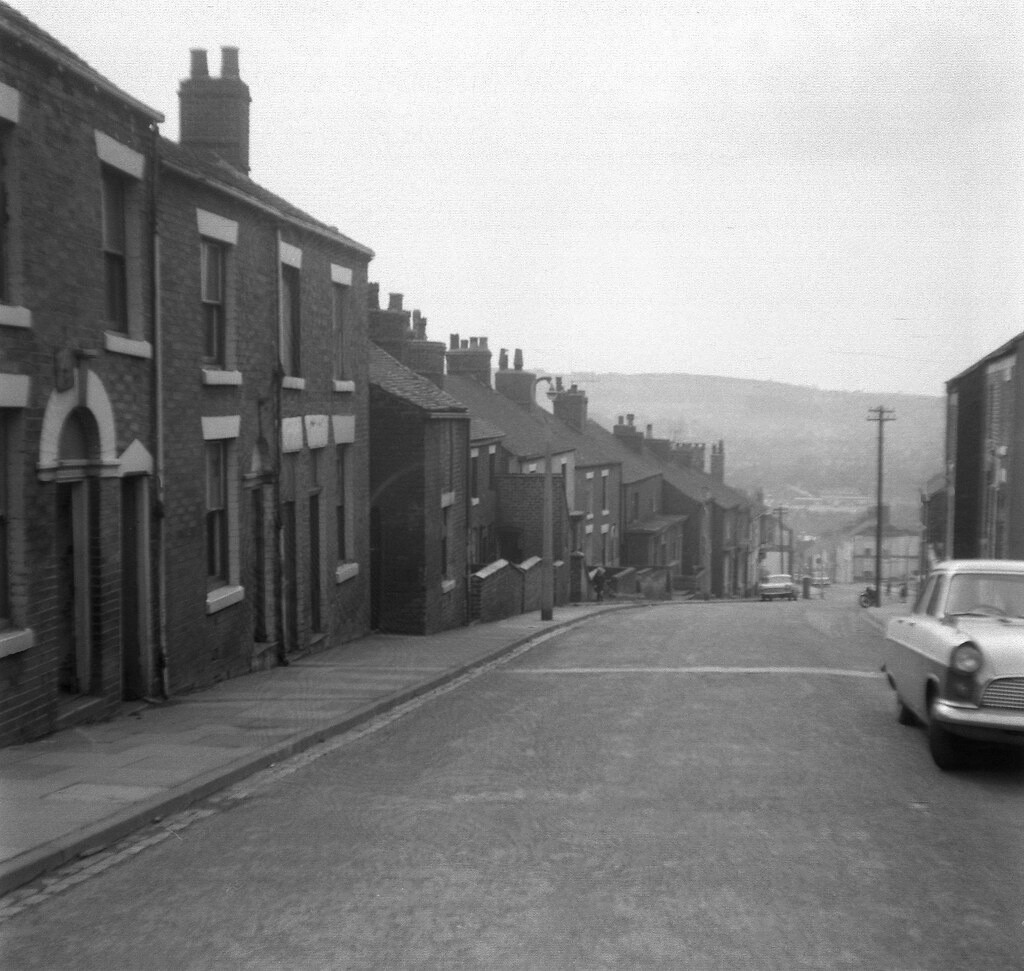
883 556 1024 774
757 572 801 601
811 570 831 586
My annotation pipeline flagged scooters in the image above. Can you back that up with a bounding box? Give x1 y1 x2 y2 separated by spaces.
859 587 877 607
